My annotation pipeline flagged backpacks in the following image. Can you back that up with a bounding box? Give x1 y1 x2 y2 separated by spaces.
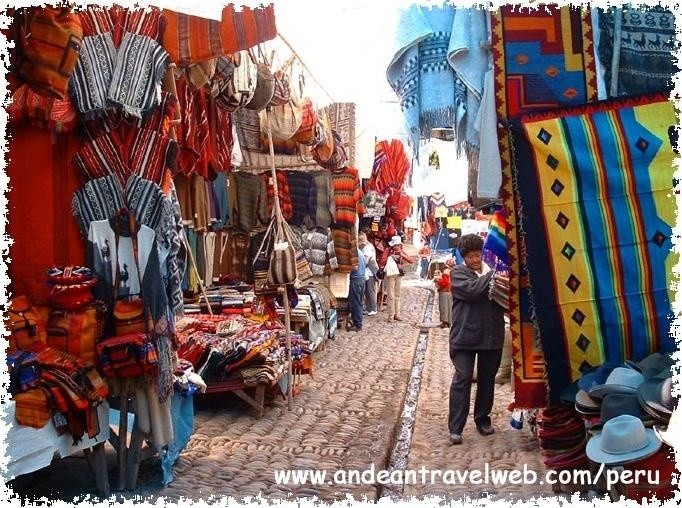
10 294 47 356
40 300 109 362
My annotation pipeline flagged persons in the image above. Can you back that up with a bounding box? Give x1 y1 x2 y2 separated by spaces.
418 243 430 280
448 233 506 444
346 249 365 331
434 259 455 329
386 221 395 237
358 232 378 316
379 235 414 322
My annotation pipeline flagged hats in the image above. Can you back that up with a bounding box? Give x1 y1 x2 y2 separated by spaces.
446 259 456 268
574 353 677 467
389 236 403 247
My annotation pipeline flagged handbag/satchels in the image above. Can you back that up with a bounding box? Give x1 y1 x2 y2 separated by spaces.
260 78 347 171
376 267 385 280
399 268 406 276
98 333 156 378
253 243 314 294
186 48 292 113
113 298 155 335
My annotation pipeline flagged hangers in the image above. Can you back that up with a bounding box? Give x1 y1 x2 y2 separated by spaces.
113 111 134 128
115 156 136 176
115 205 137 226
109 19 130 37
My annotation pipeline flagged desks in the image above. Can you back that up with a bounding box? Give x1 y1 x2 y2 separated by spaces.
0 395 111 498
220 356 290 418
276 302 312 338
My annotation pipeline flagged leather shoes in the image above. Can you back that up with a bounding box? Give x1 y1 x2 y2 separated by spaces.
395 314 404 321
362 310 369 314
347 325 362 331
476 424 494 436
450 432 463 443
436 322 449 328
388 315 395 322
368 310 377 316
